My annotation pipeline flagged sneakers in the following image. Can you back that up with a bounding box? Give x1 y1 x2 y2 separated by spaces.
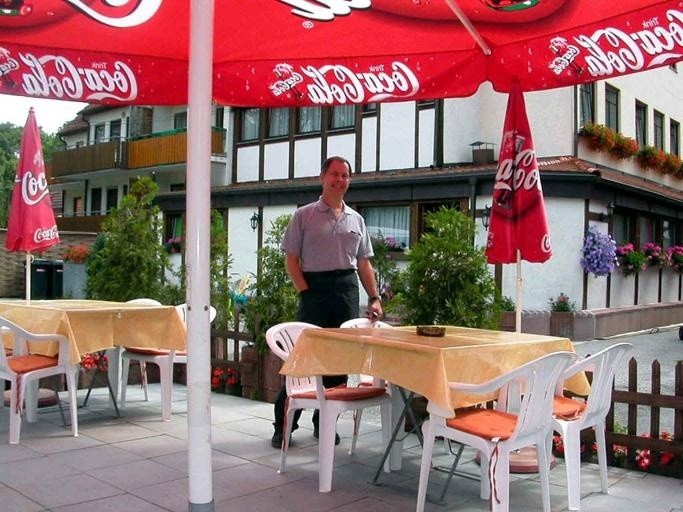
314 429 340 445
271 430 292 450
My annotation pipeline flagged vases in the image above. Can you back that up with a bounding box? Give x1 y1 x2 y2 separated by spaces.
171 247 176 254
225 381 235 394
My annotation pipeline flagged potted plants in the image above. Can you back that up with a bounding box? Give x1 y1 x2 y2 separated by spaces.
231 214 304 407
382 200 507 436
82 174 176 389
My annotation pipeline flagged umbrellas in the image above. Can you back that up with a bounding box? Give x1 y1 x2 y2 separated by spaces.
8 102 58 301
1 2 546 374
484 76 552 332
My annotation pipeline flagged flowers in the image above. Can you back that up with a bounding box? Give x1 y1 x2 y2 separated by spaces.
79 352 108 372
579 224 621 281
210 364 224 392
552 424 683 471
616 242 646 278
167 237 182 248
59 240 90 265
579 123 682 179
665 244 683 274
641 240 666 271
225 368 239 385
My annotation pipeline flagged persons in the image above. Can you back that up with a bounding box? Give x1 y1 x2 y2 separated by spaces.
271 149 384 451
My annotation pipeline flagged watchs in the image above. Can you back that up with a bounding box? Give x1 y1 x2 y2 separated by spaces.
368 295 384 305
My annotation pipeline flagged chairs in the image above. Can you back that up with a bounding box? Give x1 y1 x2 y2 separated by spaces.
1 317 80 445
107 297 167 411
414 351 578 512
266 320 393 494
117 303 220 424
339 316 425 472
510 341 635 512
